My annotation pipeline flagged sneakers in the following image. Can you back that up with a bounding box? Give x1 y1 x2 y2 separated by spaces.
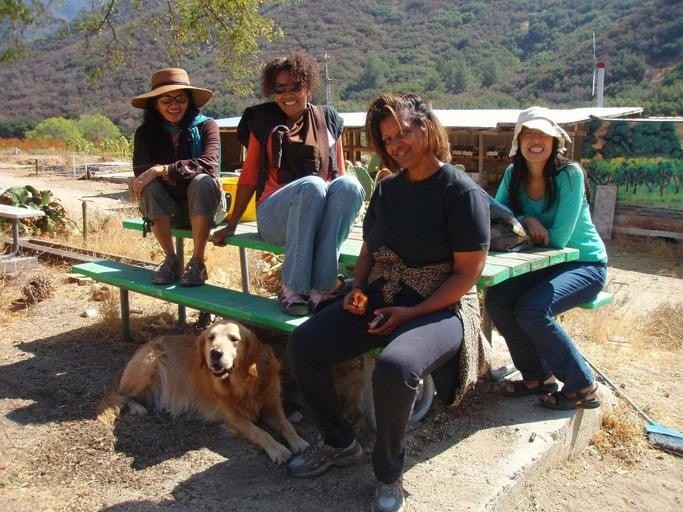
180 257 208 286
151 251 183 284
308 287 341 313
286 430 364 478
370 469 403 512
278 283 309 316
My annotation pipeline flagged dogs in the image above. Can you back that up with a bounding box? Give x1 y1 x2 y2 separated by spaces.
95 317 310 467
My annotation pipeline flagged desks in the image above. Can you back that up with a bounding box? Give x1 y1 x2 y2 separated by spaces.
120 209 580 432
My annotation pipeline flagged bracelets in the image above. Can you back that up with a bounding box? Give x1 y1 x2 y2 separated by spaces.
352 287 362 289
226 223 236 231
163 164 167 181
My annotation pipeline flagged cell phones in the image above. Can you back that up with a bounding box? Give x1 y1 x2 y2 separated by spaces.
368 312 383 329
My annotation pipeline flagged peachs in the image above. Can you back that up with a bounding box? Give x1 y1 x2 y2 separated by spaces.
353 291 368 307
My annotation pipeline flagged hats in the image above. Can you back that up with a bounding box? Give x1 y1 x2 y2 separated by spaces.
508 106 572 158
132 68 214 109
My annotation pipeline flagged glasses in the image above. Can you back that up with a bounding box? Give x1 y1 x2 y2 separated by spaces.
272 81 307 93
159 94 190 104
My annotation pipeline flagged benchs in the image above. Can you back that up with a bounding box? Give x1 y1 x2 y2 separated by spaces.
584 290 619 310
72 259 397 440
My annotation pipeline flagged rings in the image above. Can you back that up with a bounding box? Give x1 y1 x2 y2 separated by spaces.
137 180 142 184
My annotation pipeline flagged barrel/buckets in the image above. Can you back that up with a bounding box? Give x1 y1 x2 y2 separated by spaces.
221 176 256 222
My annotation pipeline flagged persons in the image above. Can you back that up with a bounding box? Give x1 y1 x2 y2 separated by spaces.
210 56 366 318
491 107 609 411
128 69 226 289
287 94 490 510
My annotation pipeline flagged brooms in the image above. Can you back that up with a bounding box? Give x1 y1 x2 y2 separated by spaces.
579 346 683 452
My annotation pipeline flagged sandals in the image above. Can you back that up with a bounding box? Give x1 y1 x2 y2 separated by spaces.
540 383 601 410
501 379 558 396
